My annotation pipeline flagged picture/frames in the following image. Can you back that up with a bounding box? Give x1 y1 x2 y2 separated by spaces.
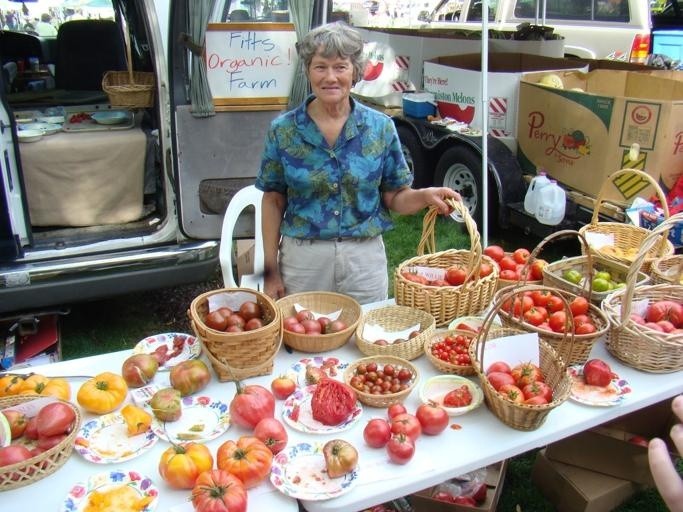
18 107 156 225
230 257 683 511
0 333 301 511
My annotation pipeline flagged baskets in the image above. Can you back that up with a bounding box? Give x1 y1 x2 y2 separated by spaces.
602 212 683 374
396 197 499 328
497 252 549 293
493 230 610 366
578 169 675 276
650 255 683 287
424 329 479 377
469 286 574 429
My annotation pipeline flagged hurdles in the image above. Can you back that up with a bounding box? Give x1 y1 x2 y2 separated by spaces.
535 74 584 92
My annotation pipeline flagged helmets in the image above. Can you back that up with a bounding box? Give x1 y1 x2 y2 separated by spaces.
132 332 201 369
14 114 64 144
463 128 485 137
286 356 353 389
280 384 361 434
74 411 156 465
565 366 629 405
270 442 357 501
148 394 232 442
62 470 160 511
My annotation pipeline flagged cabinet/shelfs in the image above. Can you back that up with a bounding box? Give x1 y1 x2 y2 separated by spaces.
406 267 445 281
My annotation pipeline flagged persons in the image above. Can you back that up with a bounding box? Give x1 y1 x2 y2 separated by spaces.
647 394 683 512
255 17 461 307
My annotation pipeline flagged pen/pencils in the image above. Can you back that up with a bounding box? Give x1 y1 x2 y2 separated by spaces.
414 0 682 70
0 0 336 320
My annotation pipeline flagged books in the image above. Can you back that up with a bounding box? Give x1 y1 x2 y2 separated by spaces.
420 50 589 141
531 448 650 511
350 26 564 107
410 455 509 511
543 393 681 486
516 66 683 204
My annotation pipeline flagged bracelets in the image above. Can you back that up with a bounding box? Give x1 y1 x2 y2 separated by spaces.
89 111 128 124
448 317 503 338
417 374 483 417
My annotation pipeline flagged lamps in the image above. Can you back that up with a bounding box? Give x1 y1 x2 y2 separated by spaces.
52 18 126 91
216 181 284 299
0 29 43 92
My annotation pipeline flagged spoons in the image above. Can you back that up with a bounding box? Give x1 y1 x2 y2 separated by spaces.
563 134 575 148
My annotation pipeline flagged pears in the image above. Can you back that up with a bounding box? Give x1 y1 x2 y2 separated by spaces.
535 74 584 92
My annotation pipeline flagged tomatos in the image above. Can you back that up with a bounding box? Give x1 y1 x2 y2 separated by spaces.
1 374 72 403
206 300 265 332
446 385 474 407
310 377 358 425
122 354 157 387
487 361 553 406
120 405 151 434
2 401 75 481
350 363 410 395
584 357 611 386
87 484 154 509
306 365 327 382
78 371 129 414
324 440 359 478
563 134 575 148
169 360 211 395
151 388 184 423
159 441 214 489
435 483 488 508
365 403 448 464
628 437 647 448
273 377 295 402
217 436 273 489
284 245 683 364
230 385 288 451
191 470 246 511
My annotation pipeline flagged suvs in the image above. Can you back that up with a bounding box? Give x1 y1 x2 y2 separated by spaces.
534 179 566 226
522 172 549 216
